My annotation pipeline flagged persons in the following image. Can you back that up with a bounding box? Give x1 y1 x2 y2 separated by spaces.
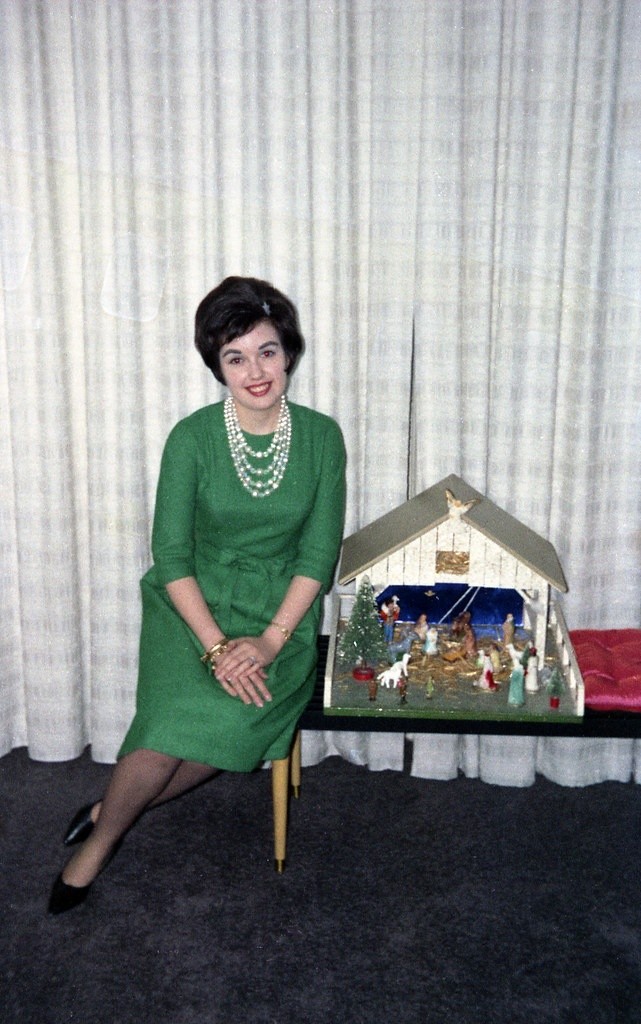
40 276 350 926
375 595 541 712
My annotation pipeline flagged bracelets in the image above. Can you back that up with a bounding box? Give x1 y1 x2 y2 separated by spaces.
202 638 230 672
270 619 291 640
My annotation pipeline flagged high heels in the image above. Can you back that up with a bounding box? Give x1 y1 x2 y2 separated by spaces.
47 800 125 914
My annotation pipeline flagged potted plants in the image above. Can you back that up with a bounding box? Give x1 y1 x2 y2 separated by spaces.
550 670 563 708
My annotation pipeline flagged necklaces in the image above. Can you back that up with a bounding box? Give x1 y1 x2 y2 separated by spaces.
223 395 292 500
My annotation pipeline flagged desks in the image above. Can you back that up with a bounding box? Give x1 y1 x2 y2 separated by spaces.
270 628 641 876
